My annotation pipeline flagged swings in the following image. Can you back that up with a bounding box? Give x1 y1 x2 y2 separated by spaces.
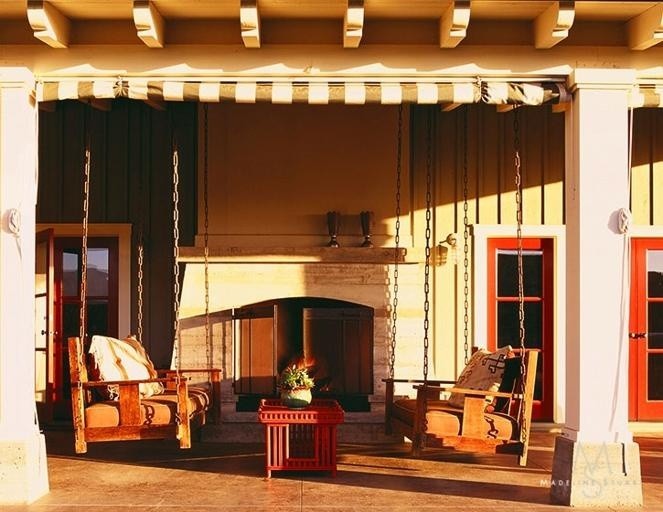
67 98 222 454
380 103 539 466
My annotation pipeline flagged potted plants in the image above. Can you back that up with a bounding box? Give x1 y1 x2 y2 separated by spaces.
280 365 314 411
